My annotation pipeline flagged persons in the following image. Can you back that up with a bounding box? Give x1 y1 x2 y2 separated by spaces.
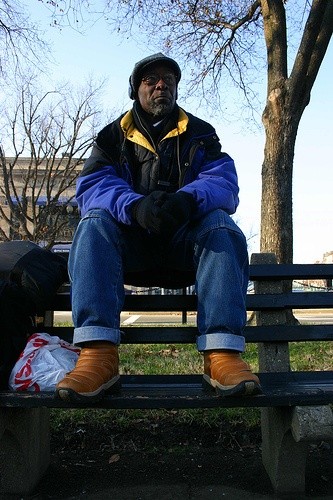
54 53 266 400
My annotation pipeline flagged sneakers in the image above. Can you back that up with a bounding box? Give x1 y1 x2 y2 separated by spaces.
54 341 121 403
202 350 263 397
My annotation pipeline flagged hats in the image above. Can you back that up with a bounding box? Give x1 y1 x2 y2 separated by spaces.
129 52 181 100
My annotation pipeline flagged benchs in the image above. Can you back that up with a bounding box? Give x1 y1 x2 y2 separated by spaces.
0 251 333 500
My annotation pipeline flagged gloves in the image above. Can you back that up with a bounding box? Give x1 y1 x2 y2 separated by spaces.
132 191 196 235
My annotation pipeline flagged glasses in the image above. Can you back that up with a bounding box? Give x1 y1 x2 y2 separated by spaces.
142 73 177 86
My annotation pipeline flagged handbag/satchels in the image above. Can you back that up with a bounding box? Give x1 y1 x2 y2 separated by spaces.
8 333 81 392
0 241 69 392
